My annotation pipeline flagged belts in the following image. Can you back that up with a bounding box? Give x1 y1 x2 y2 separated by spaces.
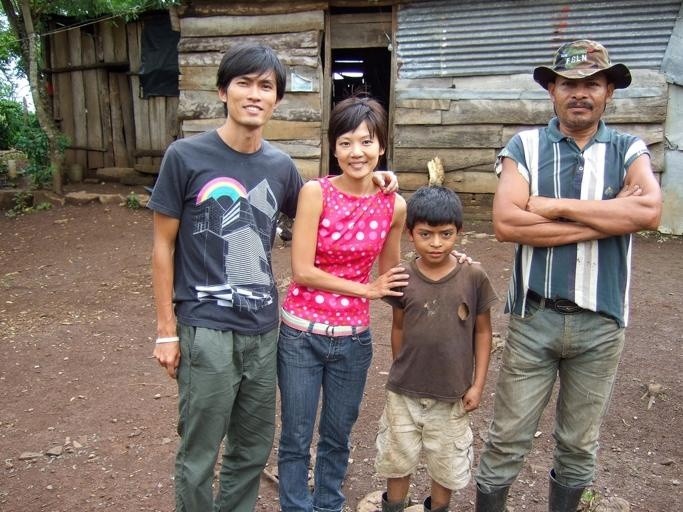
280 310 368 337
528 290 584 314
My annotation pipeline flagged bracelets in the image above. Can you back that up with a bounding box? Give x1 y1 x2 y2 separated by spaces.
156 336 179 343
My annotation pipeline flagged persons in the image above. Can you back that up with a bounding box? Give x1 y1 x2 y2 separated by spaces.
275 98 408 510
471 39 665 512
376 186 499 512
146 42 400 512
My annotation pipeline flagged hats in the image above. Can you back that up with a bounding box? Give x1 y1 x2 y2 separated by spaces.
534 40 631 90
